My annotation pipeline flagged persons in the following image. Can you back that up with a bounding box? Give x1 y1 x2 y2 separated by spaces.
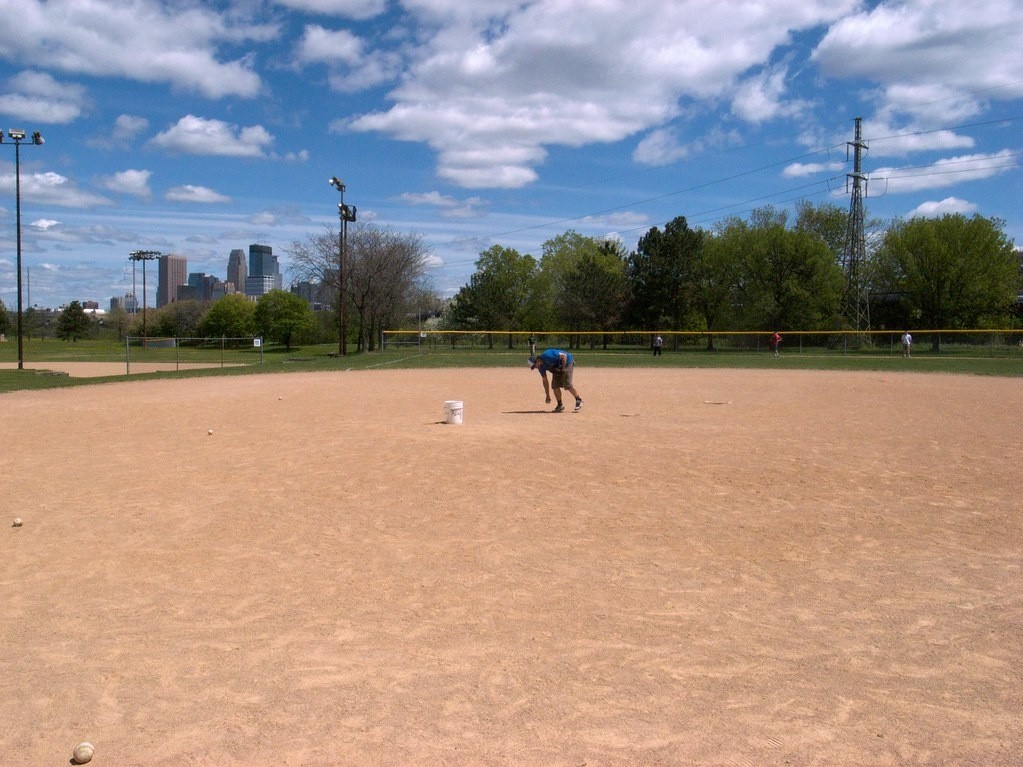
654 334 662 356
773 331 782 357
528 349 584 412
529 333 536 355
901 330 912 359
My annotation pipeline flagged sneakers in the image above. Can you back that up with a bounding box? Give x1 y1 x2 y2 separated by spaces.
574 398 584 411
552 405 565 413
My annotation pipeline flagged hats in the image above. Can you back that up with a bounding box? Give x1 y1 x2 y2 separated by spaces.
527 355 538 370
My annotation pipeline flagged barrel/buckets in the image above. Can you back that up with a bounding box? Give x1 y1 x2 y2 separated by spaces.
444 400 463 425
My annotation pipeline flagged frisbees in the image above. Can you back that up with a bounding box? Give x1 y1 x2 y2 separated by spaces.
703 400 734 405
619 413 641 417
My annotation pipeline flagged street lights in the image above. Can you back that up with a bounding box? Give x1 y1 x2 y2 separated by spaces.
128 249 163 349
328 176 346 354
0 126 46 371
336 202 358 356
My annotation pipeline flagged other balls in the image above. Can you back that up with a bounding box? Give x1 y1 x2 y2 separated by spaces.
207 429 213 435
72 741 94 764
12 517 24 526
278 396 283 400
